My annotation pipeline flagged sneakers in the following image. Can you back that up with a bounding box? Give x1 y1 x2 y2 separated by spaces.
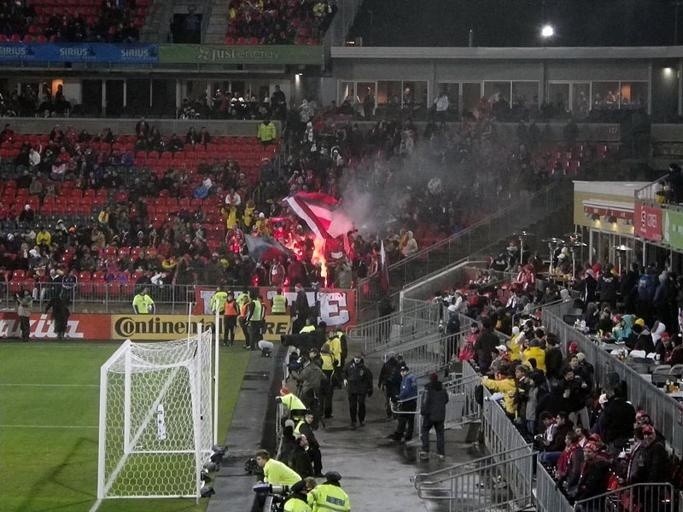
384 414 398 421
346 418 368 429
391 433 412 441
222 340 261 351
419 450 446 462
313 471 327 478
319 411 334 419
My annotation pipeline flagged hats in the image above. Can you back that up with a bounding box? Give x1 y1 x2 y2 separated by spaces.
568 341 585 362
429 372 438 382
584 433 601 452
515 358 542 384
660 332 683 345
512 320 558 346
603 307 645 333
494 343 507 351
635 410 655 433
598 394 608 404
399 365 409 372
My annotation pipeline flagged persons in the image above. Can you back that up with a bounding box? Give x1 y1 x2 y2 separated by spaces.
419 373 448 460
131 286 155 314
391 367 418 441
253 449 301 485
209 286 266 350
269 282 350 511
396 355 408 371
342 353 372 430
44 286 74 337
14 287 33 341
377 350 402 420
375 287 395 345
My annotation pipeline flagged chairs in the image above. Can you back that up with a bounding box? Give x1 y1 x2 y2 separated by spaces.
224 1 334 45
428 263 683 512
0 132 606 296
0 0 148 46
